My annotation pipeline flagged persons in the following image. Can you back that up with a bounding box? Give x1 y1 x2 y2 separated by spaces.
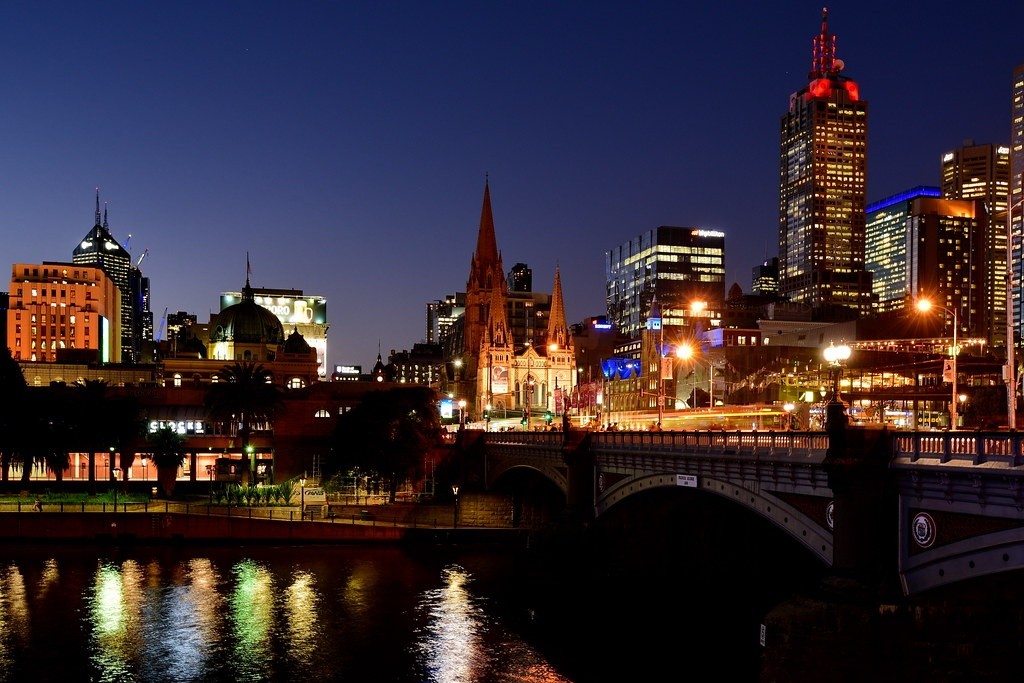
534 423 563 432
650 421 663 431
500 426 514 432
600 422 619 432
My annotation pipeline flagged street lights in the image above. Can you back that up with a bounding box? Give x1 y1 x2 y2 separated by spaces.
678 346 714 411
658 301 704 428
527 344 559 432
298 474 308 520
451 484 461 528
917 299 958 430
823 342 851 403
